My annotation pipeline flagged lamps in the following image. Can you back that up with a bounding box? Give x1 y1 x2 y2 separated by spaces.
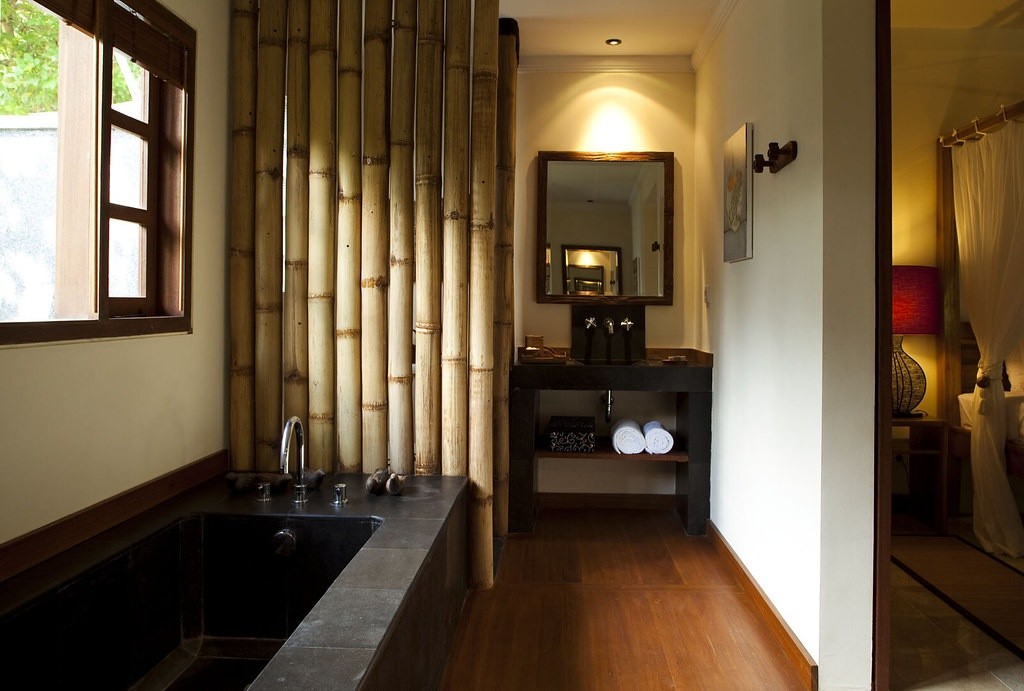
891 266 945 417
546 243 552 267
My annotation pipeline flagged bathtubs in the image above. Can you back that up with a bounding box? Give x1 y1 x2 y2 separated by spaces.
2 513 384 690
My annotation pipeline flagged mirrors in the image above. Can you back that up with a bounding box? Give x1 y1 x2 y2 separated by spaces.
536 151 674 306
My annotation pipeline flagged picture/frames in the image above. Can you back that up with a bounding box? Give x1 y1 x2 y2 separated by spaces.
722 124 753 264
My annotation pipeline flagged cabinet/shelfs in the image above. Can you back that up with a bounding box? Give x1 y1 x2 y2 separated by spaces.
891 415 949 536
510 358 711 535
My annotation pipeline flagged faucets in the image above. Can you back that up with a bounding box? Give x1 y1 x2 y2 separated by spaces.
606 319 614 335
279 414 307 504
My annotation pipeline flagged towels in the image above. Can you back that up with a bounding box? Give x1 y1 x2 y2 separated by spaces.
610 418 645 454
643 420 674 454
